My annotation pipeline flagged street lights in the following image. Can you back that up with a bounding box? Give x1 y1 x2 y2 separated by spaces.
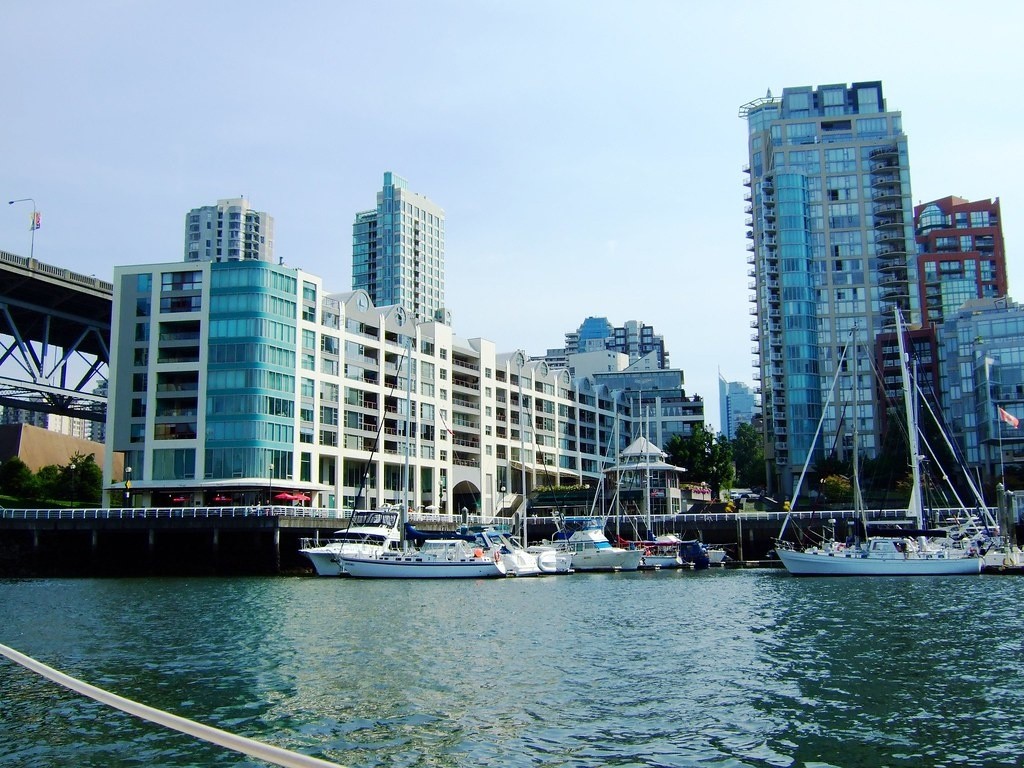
500 486 505 516
269 464 274 505
125 466 131 507
363 472 369 509
701 481 706 513
438 481 444 513
69 465 76 508
8 198 37 257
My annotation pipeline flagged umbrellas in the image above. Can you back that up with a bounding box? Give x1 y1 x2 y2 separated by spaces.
425 505 440 512
276 492 311 501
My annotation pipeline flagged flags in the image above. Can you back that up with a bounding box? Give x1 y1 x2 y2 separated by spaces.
999 407 1019 429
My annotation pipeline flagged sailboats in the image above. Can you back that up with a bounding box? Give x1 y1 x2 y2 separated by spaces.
285 295 1024 579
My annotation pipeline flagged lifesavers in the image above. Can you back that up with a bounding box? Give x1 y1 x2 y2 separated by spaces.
494 551 501 562
837 544 846 552
1002 558 1014 566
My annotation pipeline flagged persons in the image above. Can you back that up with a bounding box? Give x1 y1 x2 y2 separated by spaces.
900 534 949 560
256 500 328 518
706 543 738 561
961 529 1022 566
642 548 659 557
386 503 413 521
845 534 862 558
